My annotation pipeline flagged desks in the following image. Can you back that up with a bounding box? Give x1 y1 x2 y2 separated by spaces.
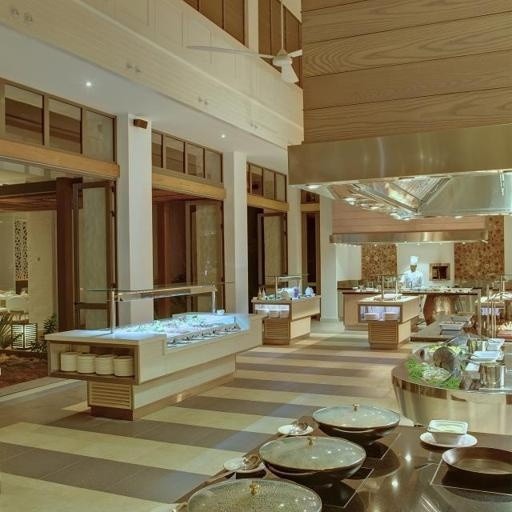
390 331 511 438
473 291 511 338
251 273 320 345
148 405 510 511
43 278 270 422
357 291 424 351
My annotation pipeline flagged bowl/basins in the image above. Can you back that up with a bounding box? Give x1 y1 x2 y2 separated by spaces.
347 441 401 480
441 447 511 483
312 403 400 445
186 477 323 511
261 435 367 489
314 481 366 511
479 363 505 387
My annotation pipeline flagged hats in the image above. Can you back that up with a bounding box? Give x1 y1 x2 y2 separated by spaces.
409 255 419 266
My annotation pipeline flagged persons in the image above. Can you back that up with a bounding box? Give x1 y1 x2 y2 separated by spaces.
398 265 424 289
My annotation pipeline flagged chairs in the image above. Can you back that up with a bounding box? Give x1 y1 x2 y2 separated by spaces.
1 287 28 320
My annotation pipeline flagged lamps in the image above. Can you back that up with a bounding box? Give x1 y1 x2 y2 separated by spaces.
9 322 38 351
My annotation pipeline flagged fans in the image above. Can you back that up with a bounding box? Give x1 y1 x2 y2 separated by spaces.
188 0 303 83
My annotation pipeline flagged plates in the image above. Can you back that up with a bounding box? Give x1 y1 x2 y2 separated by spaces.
258 308 288 318
364 312 397 321
419 432 476 448
223 456 263 474
465 337 505 378
279 423 313 437
60 351 133 376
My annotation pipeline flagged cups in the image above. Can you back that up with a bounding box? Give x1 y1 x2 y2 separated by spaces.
217 310 224 315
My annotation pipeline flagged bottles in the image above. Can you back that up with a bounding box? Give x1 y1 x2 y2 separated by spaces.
258 287 266 300
279 291 289 299
